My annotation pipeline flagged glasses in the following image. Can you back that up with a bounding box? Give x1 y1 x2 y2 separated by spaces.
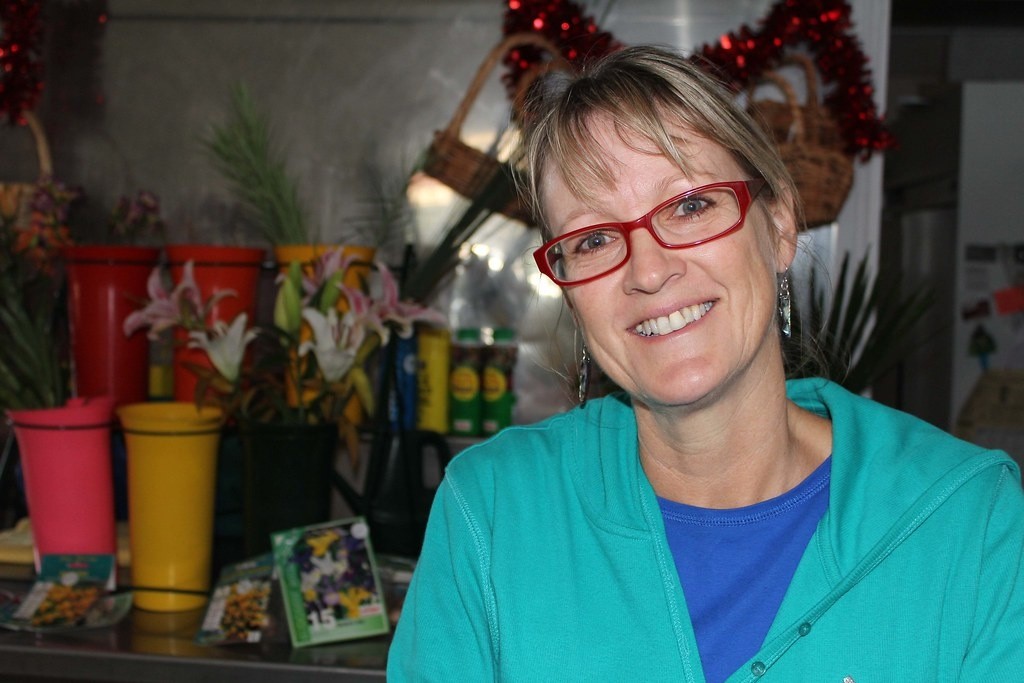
532 178 769 287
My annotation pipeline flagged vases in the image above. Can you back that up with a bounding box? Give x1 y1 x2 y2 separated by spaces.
116 401 226 613
61 245 161 405
165 244 266 403
7 398 115 594
239 421 339 561
276 245 374 409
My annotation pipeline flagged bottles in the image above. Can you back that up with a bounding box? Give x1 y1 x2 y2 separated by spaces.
449 328 519 437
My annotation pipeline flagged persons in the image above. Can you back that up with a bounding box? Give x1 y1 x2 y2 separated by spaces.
382 43 1024 683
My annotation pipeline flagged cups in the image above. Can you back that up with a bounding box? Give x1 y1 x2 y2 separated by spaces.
121 402 223 609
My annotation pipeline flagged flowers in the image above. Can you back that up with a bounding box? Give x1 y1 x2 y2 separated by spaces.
123 247 445 476
29 173 168 245
